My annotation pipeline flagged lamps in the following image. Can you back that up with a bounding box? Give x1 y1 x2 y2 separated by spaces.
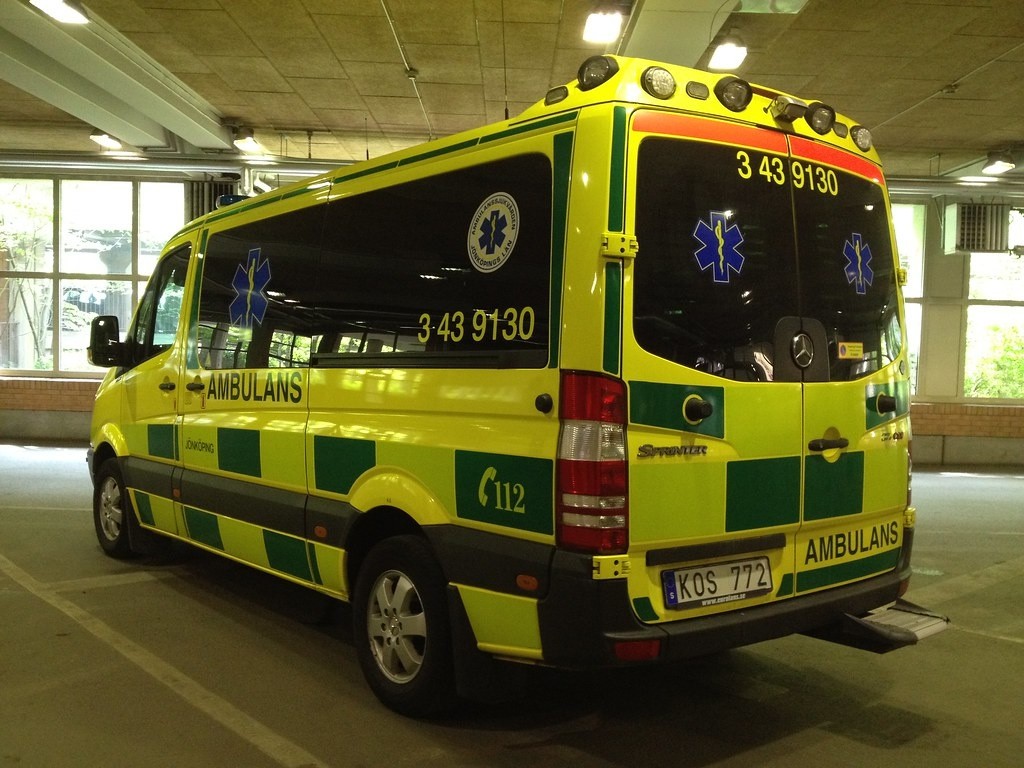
983 152 1017 177
231 127 259 152
89 128 123 150
708 33 750 71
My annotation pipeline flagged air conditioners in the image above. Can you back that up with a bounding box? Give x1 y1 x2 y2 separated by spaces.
942 202 1010 254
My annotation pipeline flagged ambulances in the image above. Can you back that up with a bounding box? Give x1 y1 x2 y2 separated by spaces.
86 53 956 723
